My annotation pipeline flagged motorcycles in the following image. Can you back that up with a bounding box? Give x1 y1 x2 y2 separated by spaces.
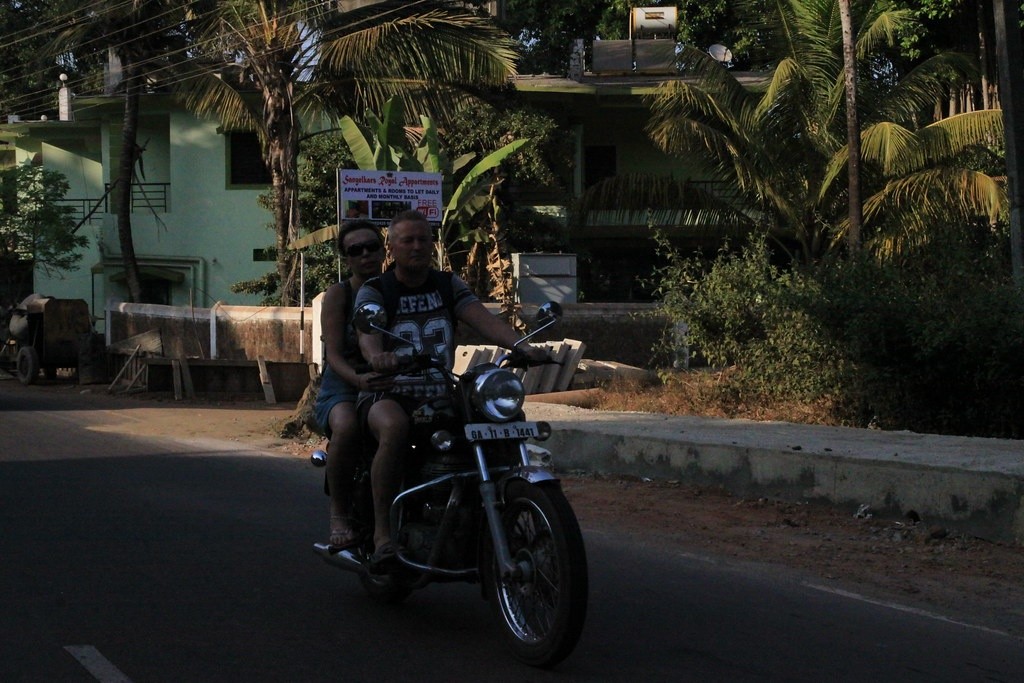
307 301 589 669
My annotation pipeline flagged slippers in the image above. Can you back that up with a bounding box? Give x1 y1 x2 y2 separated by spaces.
373 530 409 560
329 515 357 547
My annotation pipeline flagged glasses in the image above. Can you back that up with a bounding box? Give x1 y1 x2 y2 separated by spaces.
342 238 384 259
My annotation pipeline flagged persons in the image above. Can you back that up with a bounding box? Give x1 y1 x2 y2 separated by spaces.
352 210 553 572
314 218 391 550
